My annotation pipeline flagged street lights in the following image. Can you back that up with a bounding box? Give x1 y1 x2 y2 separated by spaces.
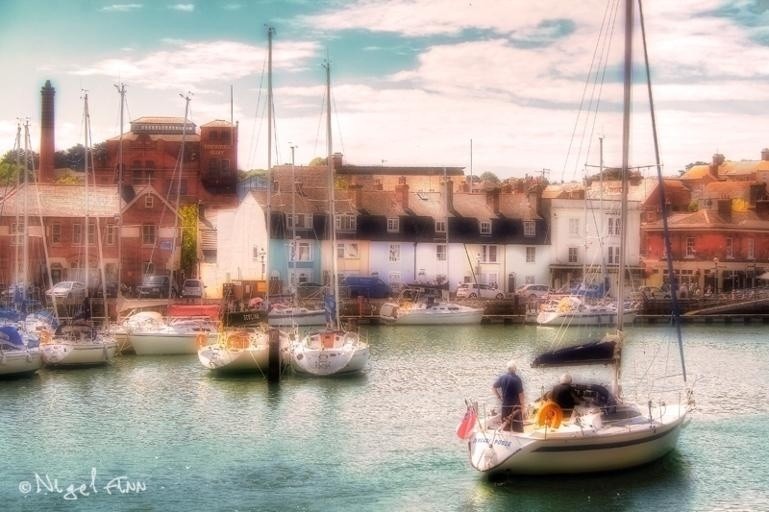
712 254 720 296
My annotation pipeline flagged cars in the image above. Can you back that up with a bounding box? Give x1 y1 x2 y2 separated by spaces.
569 282 610 298
513 283 551 297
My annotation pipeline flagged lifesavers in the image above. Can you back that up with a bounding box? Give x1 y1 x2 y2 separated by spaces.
536 402 563 430
197 334 207 347
457 402 477 438
342 332 358 346
227 335 242 353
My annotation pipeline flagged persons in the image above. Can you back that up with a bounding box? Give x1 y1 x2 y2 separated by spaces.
649 277 711 300
548 372 588 410
492 360 528 433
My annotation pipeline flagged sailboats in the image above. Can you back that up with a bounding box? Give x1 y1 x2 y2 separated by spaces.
456 0 699 482
534 135 641 330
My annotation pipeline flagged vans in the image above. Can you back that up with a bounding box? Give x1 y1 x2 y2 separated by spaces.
343 275 392 300
456 282 506 300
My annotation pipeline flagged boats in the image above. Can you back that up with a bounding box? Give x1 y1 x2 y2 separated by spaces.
380 286 487 326
